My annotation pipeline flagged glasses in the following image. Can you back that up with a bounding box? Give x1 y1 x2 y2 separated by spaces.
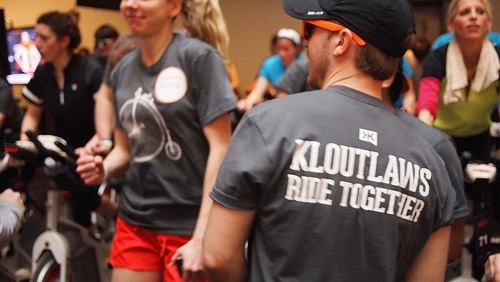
301 19 366 47
97 39 112 45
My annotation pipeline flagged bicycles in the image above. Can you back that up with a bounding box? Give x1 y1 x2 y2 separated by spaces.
0 131 116 281
440 134 500 280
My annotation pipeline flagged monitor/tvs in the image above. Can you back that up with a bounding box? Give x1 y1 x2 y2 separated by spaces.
6 26 42 84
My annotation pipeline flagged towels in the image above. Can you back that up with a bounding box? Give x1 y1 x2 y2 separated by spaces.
443 37 500 106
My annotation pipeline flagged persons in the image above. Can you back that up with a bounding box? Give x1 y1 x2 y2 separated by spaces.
0 77 12 126
20 11 119 230
471 169 500 282
415 0 500 161
14 30 41 75
278 57 313 95
93 25 119 67
181 0 230 64
203 0 457 282
77 0 238 282
0 187 25 239
382 62 471 260
237 28 303 111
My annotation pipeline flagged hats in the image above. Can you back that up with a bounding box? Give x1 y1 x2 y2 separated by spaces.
284 0 416 58
